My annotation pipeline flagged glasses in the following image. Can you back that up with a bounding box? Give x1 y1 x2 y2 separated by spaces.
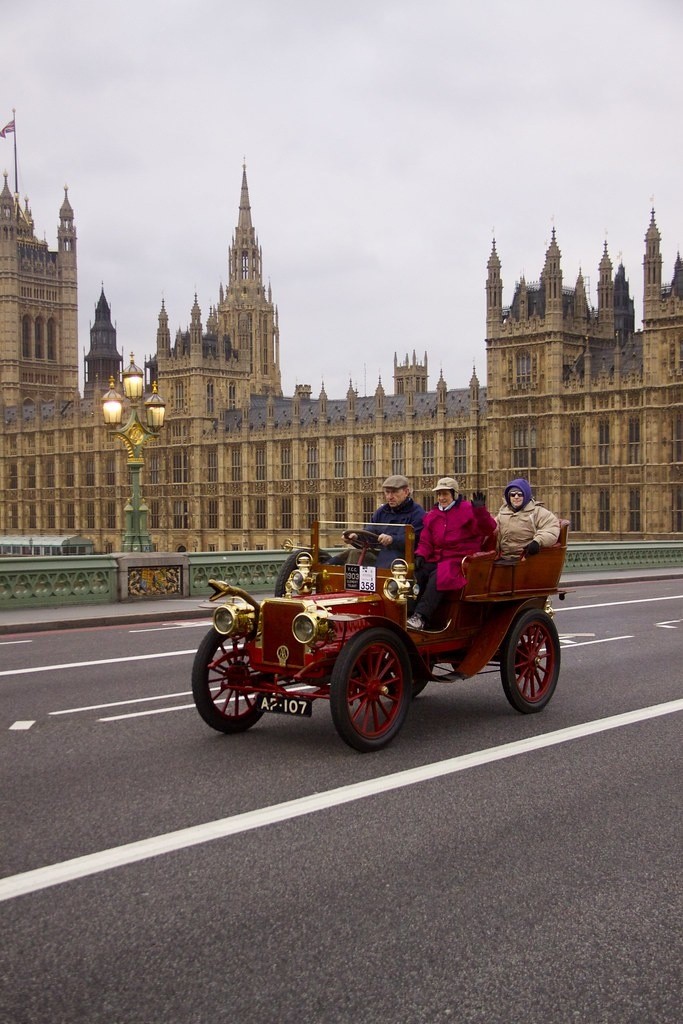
510 492 524 497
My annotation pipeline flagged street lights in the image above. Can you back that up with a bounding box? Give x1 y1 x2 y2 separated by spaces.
100 351 167 552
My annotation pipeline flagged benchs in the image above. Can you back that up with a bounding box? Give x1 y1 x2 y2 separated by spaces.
493 519 571 595
445 516 499 596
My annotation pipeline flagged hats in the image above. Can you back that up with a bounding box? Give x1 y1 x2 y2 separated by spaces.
432 477 459 493
382 474 409 488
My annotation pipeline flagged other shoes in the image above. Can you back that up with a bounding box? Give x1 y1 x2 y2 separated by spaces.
406 611 425 631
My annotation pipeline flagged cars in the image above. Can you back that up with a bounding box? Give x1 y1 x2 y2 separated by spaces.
191 519 576 752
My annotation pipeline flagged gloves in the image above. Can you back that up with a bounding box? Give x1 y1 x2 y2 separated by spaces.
415 555 425 572
523 540 540 558
470 490 486 506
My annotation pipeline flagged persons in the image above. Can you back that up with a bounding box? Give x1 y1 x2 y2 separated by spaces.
407 477 497 631
334 474 425 570
494 479 560 563
